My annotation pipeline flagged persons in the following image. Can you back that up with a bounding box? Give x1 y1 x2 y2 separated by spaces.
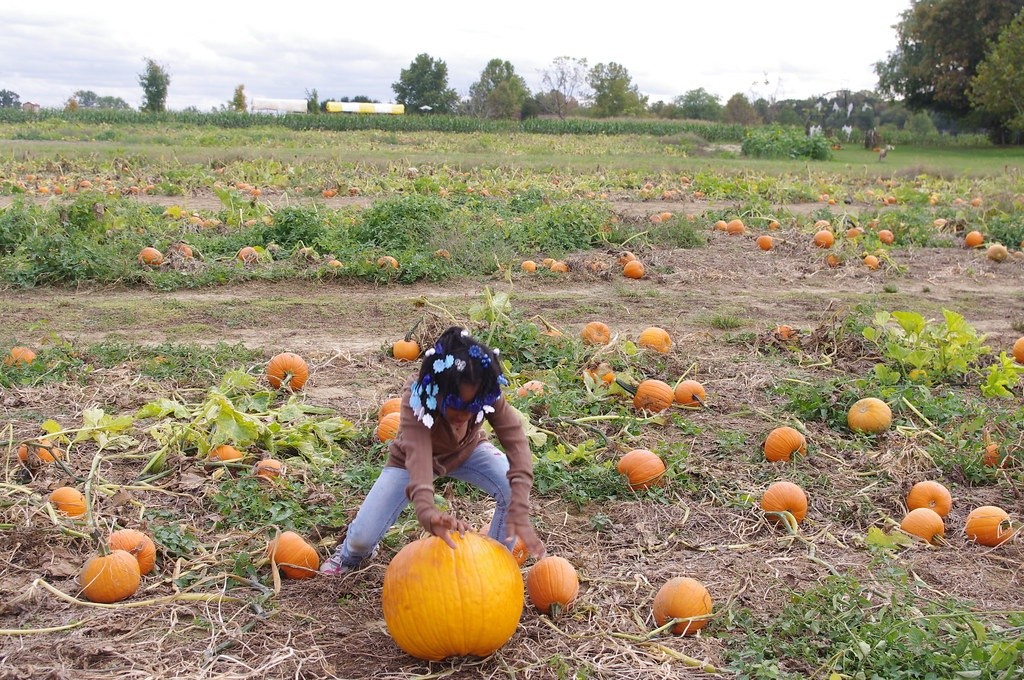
316 325 544 578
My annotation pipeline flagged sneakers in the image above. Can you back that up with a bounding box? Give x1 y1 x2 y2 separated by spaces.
320 544 350 576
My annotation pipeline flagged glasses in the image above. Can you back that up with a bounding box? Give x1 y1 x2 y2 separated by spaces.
444 393 482 414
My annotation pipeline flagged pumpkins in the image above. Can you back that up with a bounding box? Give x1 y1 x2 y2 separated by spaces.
0 171 1024 638
383 529 524 662
